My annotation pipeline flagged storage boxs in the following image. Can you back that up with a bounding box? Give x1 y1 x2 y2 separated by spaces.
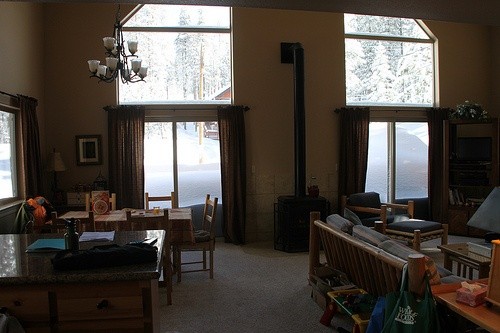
307 265 354 310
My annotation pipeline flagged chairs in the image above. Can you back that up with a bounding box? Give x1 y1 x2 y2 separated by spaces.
339 192 414 223
24 189 218 279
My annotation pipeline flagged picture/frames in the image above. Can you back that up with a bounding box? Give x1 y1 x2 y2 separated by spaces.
75 134 102 166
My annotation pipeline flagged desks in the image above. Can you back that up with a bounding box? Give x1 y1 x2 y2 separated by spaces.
56 208 196 281
437 241 493 279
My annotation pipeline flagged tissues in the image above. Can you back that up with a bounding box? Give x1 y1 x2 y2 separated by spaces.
454 275 488 308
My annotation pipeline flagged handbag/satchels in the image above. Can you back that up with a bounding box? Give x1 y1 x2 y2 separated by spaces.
380 263 440 333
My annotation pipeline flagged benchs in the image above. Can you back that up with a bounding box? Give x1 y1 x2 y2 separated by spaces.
306 210 468 332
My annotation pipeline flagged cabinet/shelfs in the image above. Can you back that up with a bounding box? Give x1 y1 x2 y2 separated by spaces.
438 119 500 237
0 228 167 333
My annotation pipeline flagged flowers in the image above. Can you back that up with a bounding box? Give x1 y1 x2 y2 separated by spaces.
449 99 488 119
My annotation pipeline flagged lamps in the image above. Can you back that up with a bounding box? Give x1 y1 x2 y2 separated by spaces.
87 4 148 84
468 185 500 308
46 148 64 204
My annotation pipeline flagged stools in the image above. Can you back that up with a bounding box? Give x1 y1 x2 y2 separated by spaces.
375 220 448 253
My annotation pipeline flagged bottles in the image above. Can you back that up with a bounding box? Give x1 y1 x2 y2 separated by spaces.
63 217 80 250
154 207 160 215
